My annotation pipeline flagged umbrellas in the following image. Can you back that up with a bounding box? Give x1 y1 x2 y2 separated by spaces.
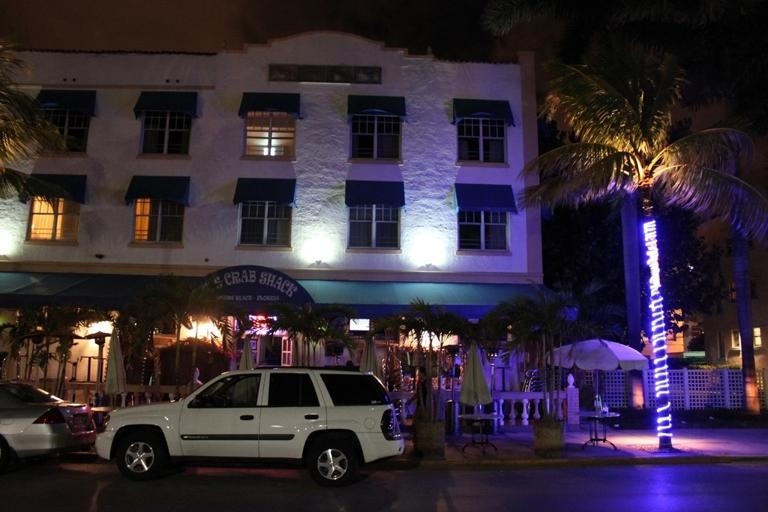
545 333 650 411
231 333 260 402
103 325 127 408
458 337 495 439
358 331 378 376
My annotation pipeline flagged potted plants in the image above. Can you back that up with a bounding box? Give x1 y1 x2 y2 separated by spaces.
479 281 586 451
363 297 473 458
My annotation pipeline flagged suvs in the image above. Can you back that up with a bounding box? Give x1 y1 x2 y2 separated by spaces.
93 363 407 485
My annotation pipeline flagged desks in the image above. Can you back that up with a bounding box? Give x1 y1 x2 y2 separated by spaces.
457 414 503 458
579 411 620 451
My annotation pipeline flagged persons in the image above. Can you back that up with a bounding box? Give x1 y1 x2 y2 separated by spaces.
187 366 202 385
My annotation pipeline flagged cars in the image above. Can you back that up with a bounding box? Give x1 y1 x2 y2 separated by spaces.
1 381 97 470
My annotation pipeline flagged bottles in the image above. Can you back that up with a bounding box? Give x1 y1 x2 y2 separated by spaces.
594 394 602 414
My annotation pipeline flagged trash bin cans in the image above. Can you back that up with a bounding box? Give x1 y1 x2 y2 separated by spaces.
38 377 71 401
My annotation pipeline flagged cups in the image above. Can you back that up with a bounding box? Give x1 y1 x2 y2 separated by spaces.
475 404 484 416
601 401 609 414
